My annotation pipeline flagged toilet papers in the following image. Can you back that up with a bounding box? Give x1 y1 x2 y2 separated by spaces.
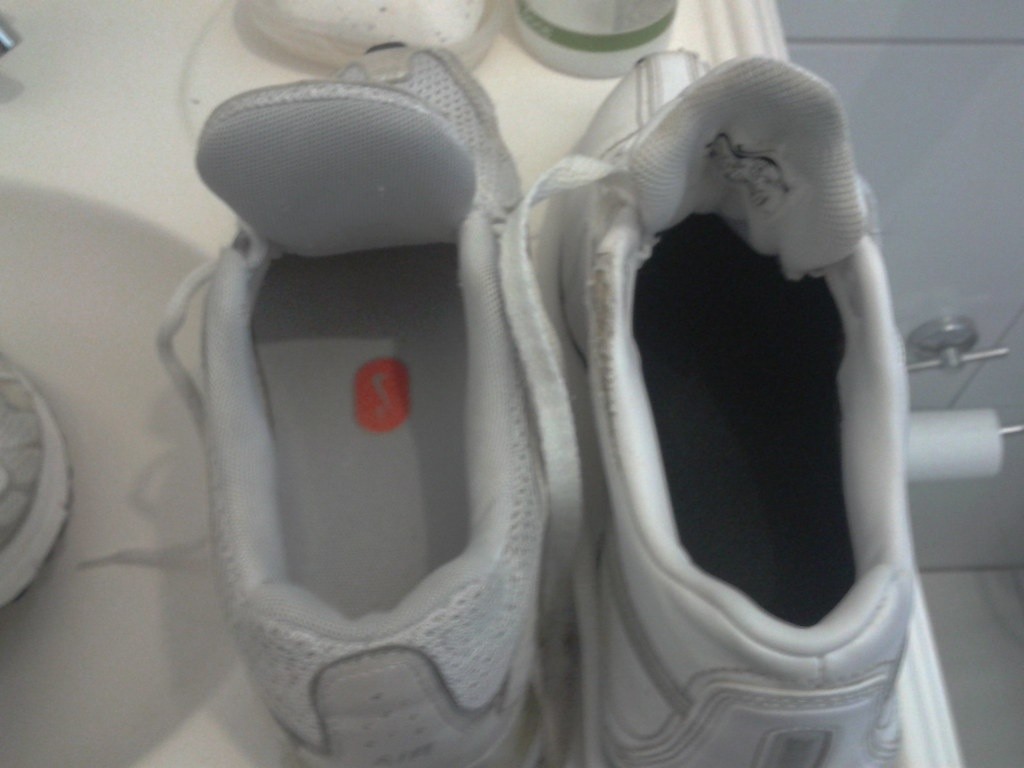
907 403 1006 485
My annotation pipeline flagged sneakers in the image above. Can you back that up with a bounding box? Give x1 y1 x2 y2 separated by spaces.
499 47 915 768
154 40 555 767
